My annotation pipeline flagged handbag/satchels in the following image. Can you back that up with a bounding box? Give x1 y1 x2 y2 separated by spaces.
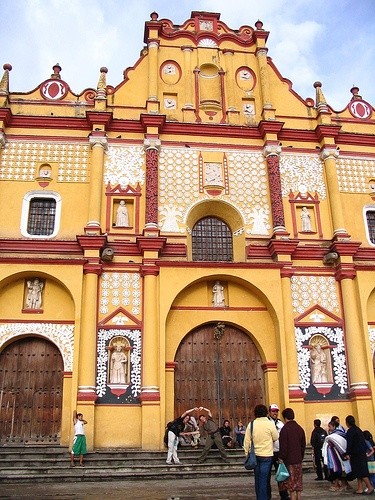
245 449 257 470
275 463 290 482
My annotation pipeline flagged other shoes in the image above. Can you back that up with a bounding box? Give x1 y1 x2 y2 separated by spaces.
281 497 290 500
225 460 230 463
354 490 363 494
366 489 374 495
70 464 75 467
315 477 323 480
195 461 201 464
329 487 336 492
167 462 172 464
79 464 84 467
175 462 183 465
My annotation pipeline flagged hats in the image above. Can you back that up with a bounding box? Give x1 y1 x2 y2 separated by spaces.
269 404 279 411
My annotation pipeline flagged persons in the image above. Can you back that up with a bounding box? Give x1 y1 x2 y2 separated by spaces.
300 207 313 232
243 404 278 500
180 416 205 448
278 407 306 500
196 406 230 464
211 280 226 306
235 419 246 448
69 410 88 466
310 415 375 495
110 341 128 383
165 406 200 465
266 404 291 500
26 278 44 309
218 420 233 448
310 342 328 383
117 200 130 227
